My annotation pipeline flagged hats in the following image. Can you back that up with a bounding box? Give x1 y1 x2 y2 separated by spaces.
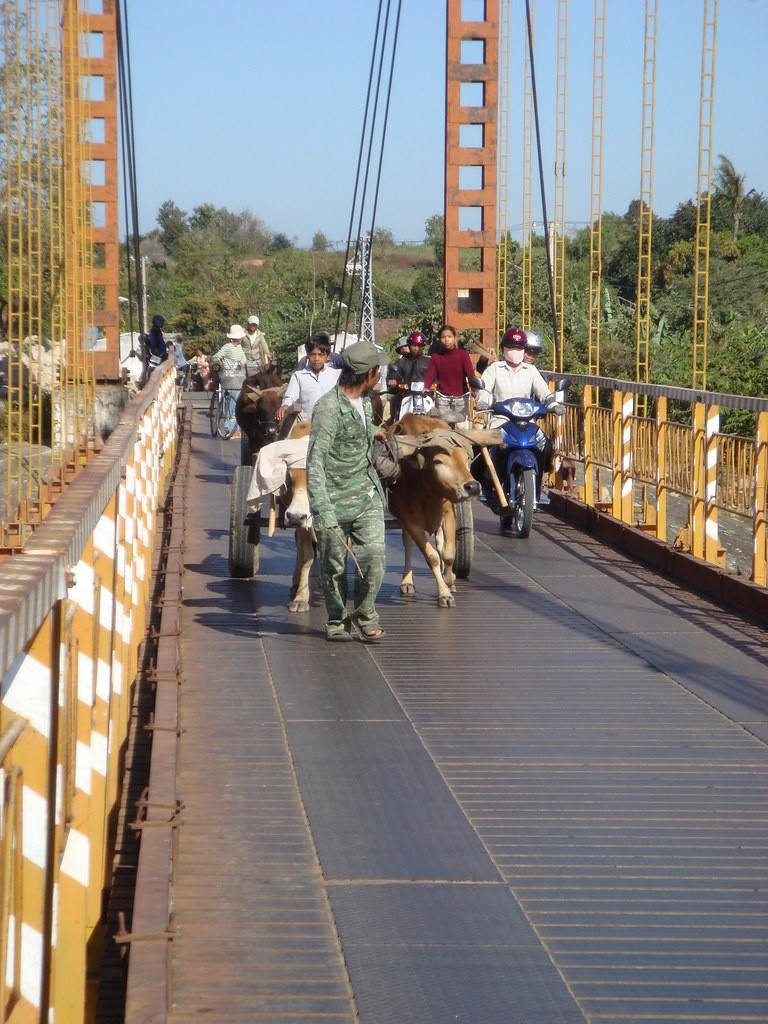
227 325 247 339
342 341 391 375
247 315 259 326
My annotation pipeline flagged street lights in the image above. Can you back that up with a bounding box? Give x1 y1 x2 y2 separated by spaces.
336 301 356 331
118 296 139 323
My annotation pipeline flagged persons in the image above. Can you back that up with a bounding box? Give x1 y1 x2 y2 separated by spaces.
166 341 188 392
380 331 438 426
274 331 343 423
187 346 212 391
422 325 476 430
475 329 566 504
306 340 387 641
204 325 247 439
128 314 168 387
241 316 272 377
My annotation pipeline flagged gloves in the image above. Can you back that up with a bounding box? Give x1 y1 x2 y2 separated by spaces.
552 405 566 415
476 412 488 424
212 361 221 372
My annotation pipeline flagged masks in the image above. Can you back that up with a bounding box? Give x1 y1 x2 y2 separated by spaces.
504 347 525 365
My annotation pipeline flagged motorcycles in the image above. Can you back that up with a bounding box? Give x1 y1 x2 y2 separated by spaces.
470 376 571 539
143 337 170 385
389 363 437 422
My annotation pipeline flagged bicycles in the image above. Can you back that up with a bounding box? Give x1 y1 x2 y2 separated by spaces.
422 385 474 431
209 358 245 440
184 362 199 392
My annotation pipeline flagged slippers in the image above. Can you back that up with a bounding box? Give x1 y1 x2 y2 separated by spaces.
359 623 386 639
325 624 354 641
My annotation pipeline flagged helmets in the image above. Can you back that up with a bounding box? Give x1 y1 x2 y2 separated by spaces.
502 329 528 350
395 336 411 355
152 315 168 326
524 330 543 352
407 332 427 347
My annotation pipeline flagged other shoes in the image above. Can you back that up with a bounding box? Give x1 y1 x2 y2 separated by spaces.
225 431 241 440
538 492 550 504
480 494 488 501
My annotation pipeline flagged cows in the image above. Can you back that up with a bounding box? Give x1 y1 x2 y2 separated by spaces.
234 373 503 614
